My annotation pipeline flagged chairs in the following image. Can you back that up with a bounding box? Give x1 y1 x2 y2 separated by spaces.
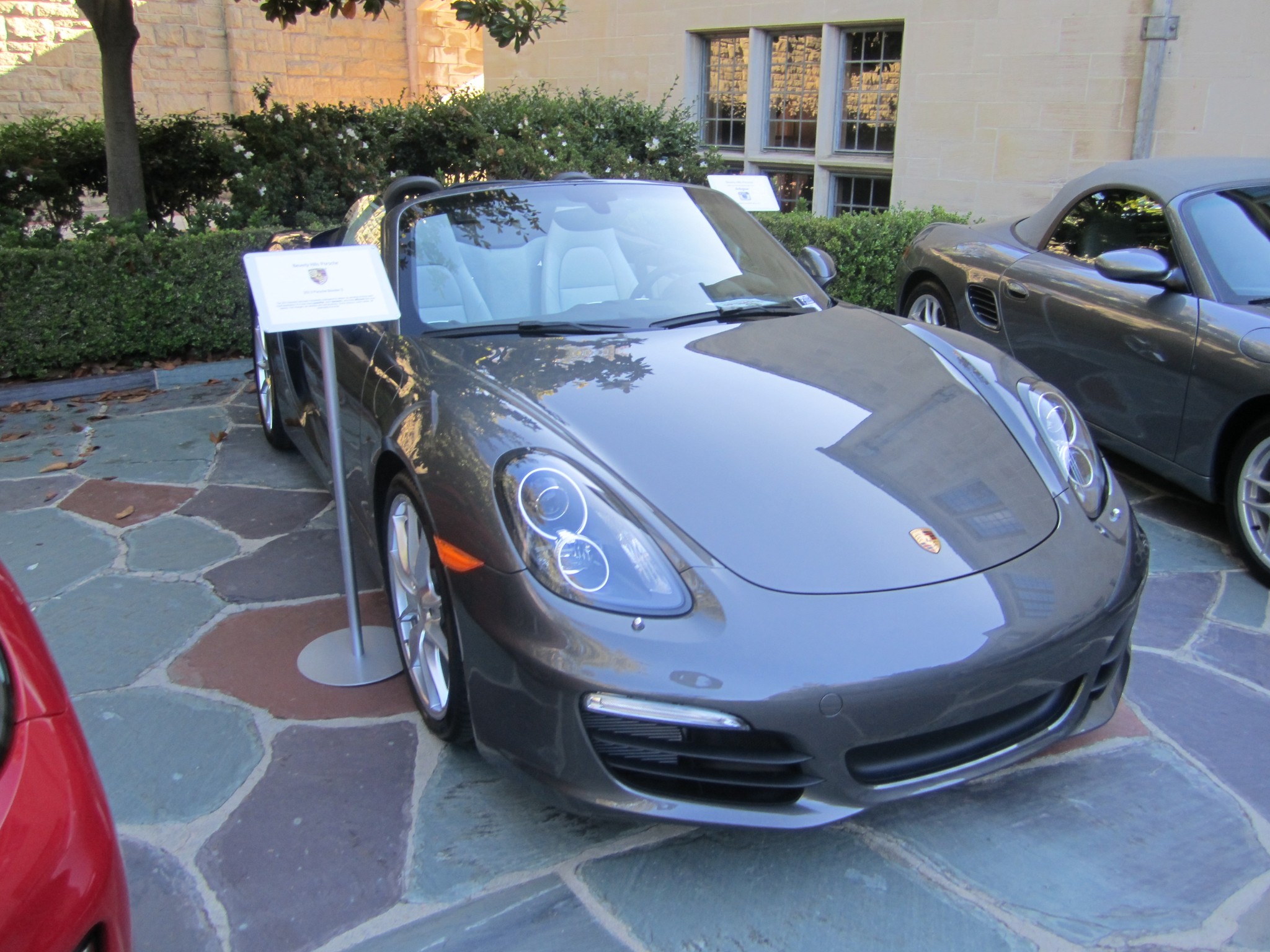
539 170 648 317
1080 210 1140 257
384 176 494 325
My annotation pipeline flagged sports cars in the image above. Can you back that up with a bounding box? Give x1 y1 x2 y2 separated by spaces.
249 163 1150 832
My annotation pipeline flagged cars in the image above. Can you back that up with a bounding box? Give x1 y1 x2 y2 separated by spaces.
896 156 1270 589
0 562 136 952
